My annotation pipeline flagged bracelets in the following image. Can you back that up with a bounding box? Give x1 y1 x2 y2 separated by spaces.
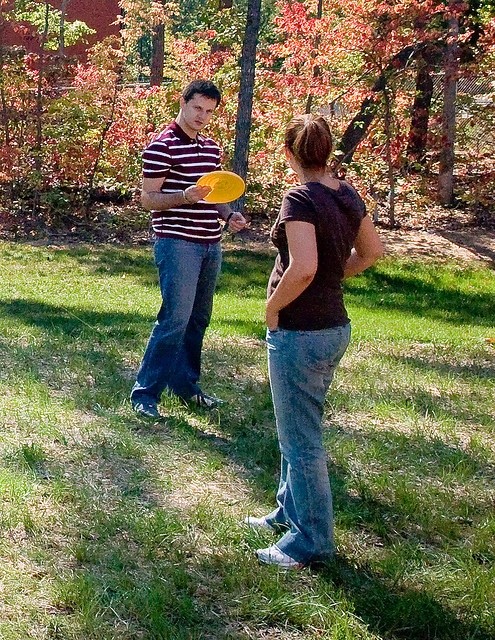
227 212 235 223
182 191 194 205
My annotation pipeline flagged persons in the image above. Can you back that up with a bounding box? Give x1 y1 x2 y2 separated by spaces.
244 113 383 567
130 79 246 422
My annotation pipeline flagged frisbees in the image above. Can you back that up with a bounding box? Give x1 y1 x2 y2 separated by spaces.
196 171 245 204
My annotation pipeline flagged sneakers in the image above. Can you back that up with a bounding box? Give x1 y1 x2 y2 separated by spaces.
178 393 225 410
129 397 165 423
254 544 334 572
244 515 288 533
